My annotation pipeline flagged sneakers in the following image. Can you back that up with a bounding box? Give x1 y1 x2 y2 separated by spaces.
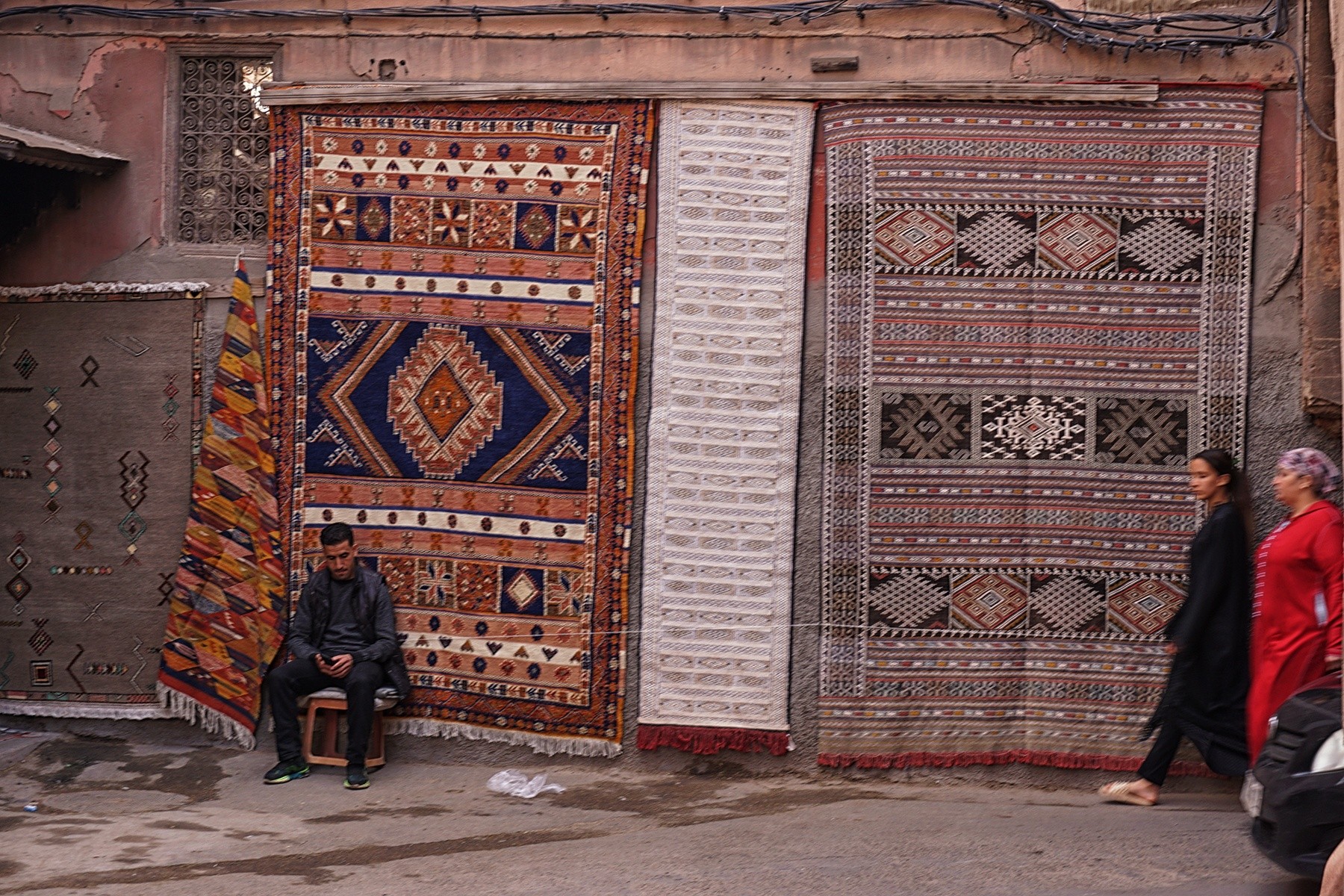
344 764 370 789
262 757 310 783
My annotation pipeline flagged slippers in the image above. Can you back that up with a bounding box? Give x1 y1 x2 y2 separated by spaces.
1099 780 1155 806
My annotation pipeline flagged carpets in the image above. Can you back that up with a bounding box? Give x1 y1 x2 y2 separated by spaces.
152 83 1266 778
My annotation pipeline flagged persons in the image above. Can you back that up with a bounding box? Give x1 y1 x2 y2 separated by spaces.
1094 448 1251 807
1318 839 1344 896
1250 448 1343 762
261 521 399 790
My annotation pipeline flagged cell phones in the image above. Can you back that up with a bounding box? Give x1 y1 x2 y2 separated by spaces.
321 654 334 666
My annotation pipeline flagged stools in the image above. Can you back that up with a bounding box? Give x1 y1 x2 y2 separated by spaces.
301 698 387 770
